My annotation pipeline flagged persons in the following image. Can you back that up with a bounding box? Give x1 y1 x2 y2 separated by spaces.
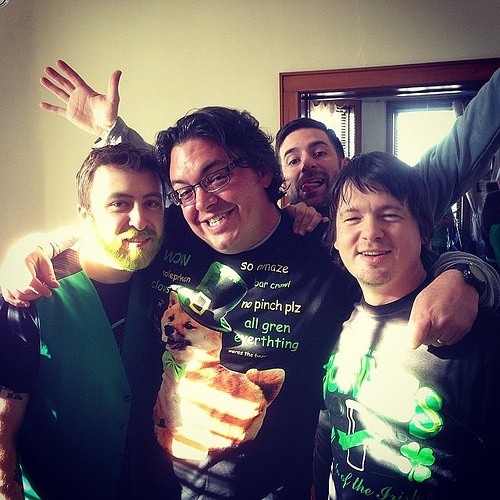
0 106 500 500
39 59 500 258
309 151 500 500
0 142 329 500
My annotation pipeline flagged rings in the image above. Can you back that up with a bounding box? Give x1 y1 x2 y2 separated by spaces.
437 338 448 345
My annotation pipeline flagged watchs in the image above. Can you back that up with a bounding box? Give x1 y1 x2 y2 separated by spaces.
450 262 489 298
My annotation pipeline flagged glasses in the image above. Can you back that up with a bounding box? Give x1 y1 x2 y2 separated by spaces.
165 153 250 207
